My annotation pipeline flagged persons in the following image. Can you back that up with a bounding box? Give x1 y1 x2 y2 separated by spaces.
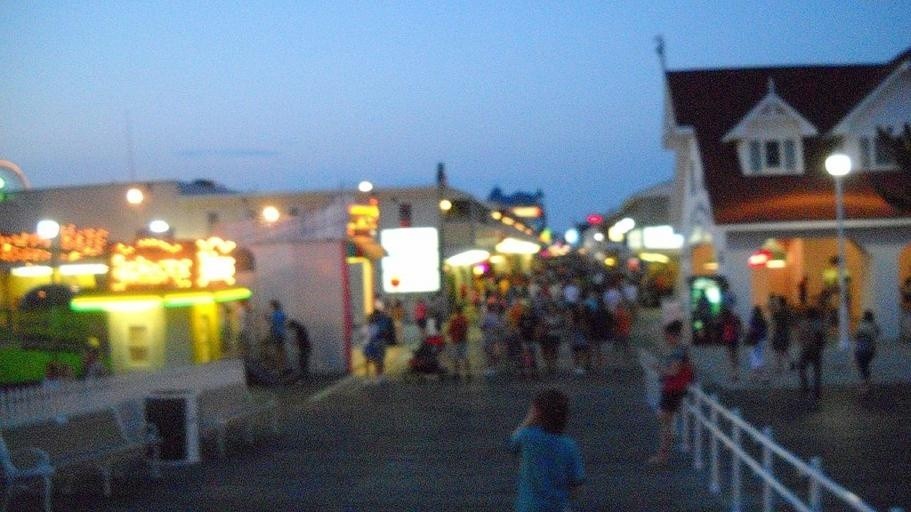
355 250 853 385
43 361 77 435
80 345 106 380
793 307 827 405
262 299 293 374
234 297 259 363
648 319 695 468
854 308 883 393
507 386 587 512
287 320 313 388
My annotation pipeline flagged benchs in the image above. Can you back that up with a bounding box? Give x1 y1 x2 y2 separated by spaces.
196 381 279 459
0 405 169 512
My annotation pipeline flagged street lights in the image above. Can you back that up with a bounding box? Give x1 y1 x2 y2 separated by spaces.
824 148 856 369
